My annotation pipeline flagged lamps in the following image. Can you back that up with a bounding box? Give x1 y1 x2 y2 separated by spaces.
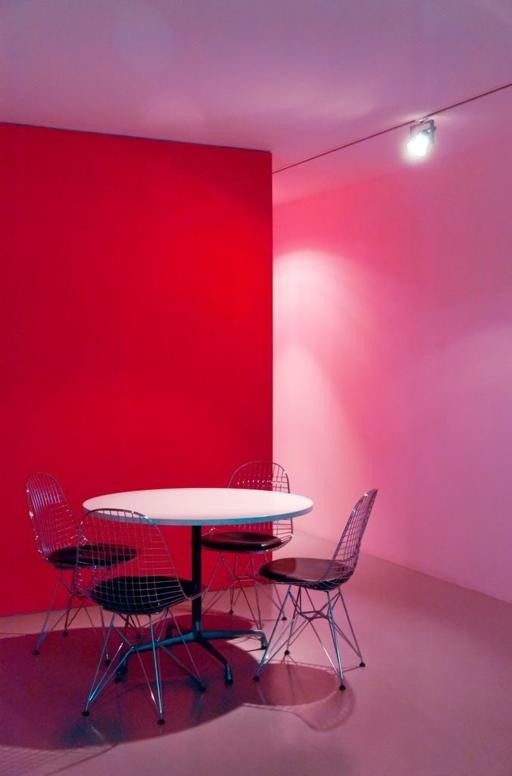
390 118 443 158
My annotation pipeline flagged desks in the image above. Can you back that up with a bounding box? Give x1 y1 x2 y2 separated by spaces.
75 486 313 686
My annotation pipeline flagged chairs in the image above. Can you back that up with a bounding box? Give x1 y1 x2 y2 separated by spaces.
251 486 381 690
72 502 206 727
21 466 139 666
195 453 294 631
